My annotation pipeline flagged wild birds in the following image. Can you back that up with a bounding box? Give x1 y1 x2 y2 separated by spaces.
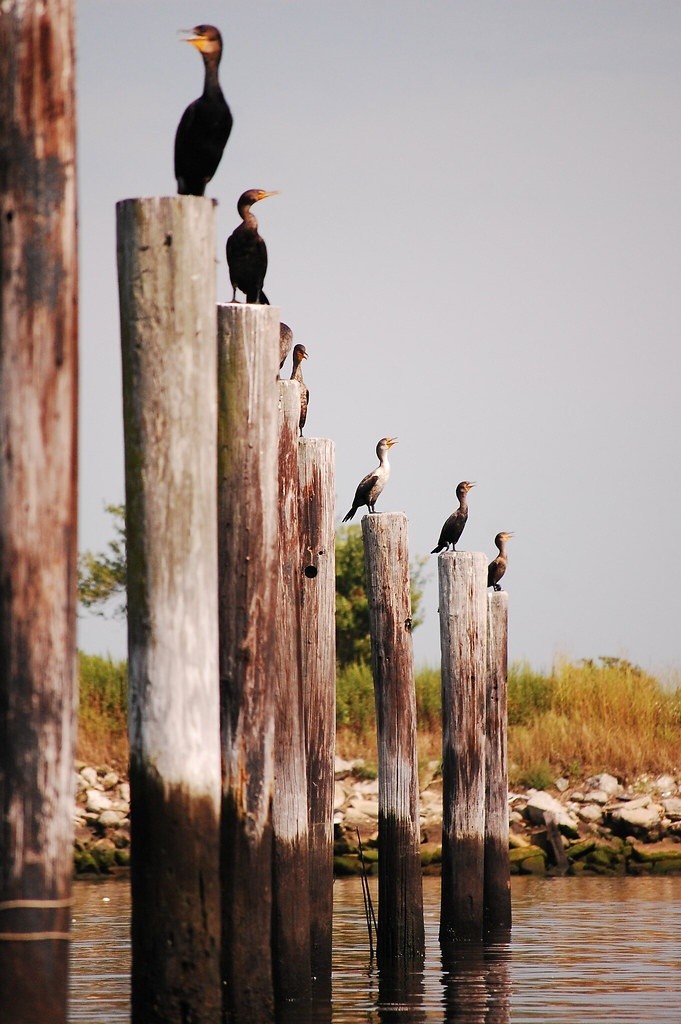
289 343 310 437
172 23 234 197
225 189 283 305
430 480 477 555
275 321 294 370
341 436 400 523
486 531 516 592
